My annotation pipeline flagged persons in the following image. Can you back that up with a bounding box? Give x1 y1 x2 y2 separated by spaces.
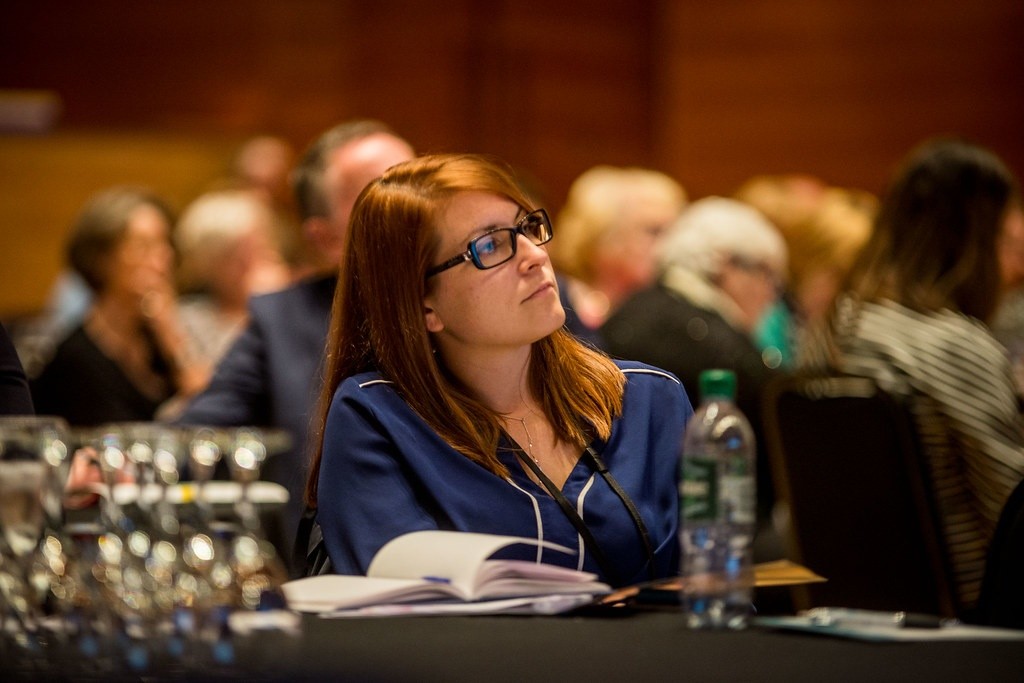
32 121 876 528
788 141 1024 605
305 153 694 589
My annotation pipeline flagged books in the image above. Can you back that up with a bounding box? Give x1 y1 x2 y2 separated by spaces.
280 529 614 613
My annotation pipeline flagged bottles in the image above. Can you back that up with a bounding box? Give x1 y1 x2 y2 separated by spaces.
679 369 757 632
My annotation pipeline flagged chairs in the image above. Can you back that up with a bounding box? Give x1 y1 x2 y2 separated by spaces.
774 363 995 623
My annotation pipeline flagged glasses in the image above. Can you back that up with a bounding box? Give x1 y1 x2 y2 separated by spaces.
424 209 554 271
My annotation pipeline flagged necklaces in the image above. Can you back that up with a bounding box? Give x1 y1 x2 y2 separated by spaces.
503 403 541 468
